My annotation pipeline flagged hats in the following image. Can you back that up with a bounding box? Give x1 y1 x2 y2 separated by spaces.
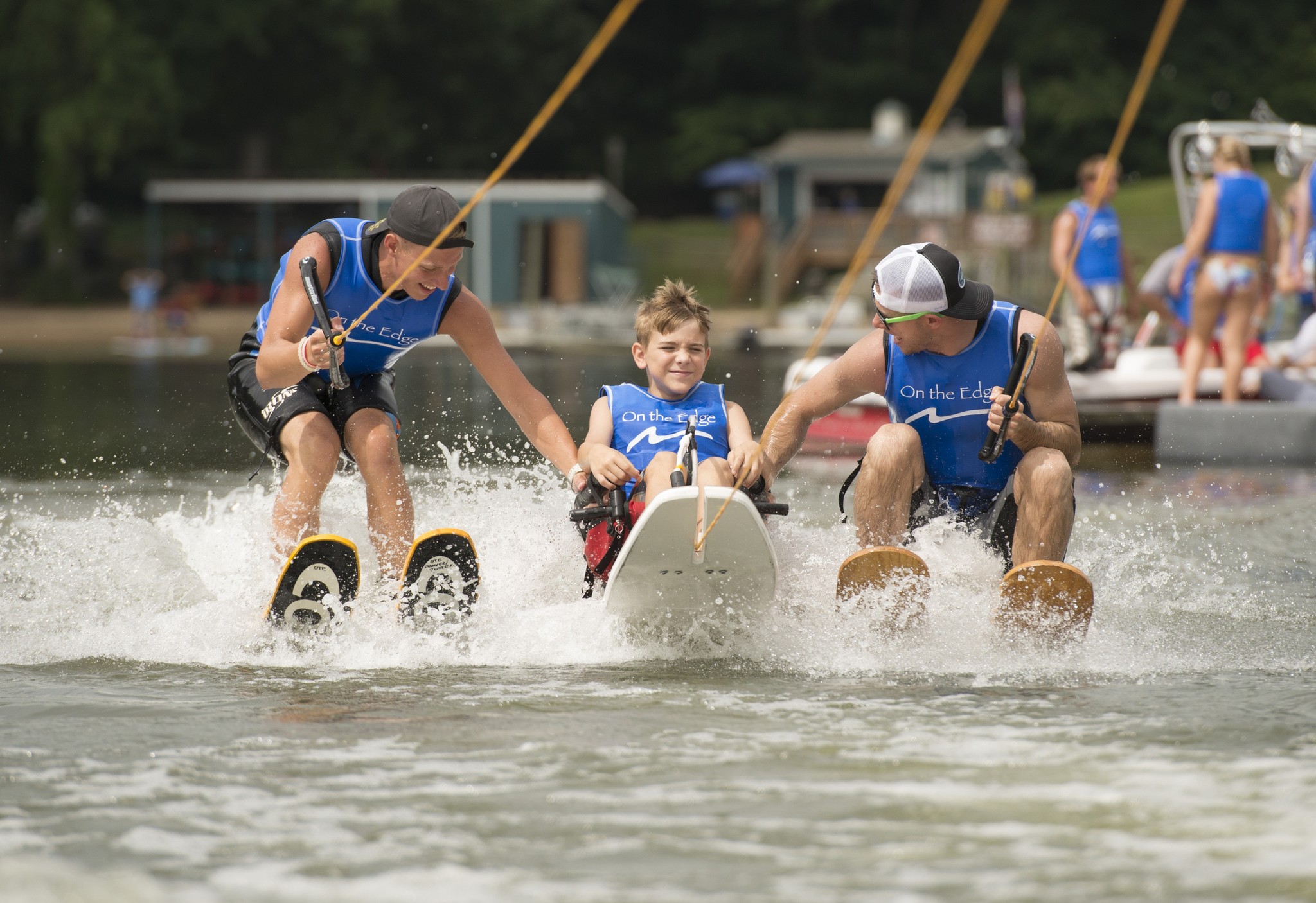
365 183 475 250
873 242 994 321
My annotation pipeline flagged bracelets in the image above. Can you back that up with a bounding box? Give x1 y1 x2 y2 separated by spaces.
298 335 321 372
567 463 582 493
302 337 319 368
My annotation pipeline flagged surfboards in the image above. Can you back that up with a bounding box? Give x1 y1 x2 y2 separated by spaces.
835 544 933 631
263 528 483 637
1003 560 1097 640
599 484 780 659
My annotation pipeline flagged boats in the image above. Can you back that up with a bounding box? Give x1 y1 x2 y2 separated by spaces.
1065 346 1301 401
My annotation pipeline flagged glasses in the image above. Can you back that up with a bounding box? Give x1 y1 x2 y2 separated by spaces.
871 281 945 330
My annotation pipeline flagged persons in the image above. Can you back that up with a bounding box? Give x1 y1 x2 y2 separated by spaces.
577 277 763 508
1140 240 1272 369
1050 153 1122 368
760 241 1080 569
1277 162 1316 317
226 186 589 595
1169 140 1280 404
